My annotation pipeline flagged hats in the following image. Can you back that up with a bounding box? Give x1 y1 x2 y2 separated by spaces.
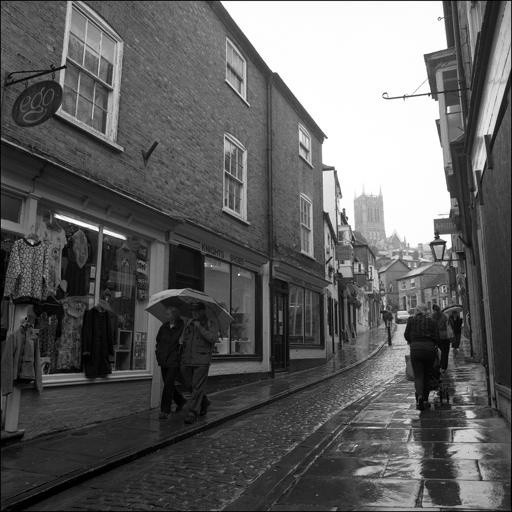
189 301 205 311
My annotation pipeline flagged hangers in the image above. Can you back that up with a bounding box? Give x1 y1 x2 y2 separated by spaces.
1 211 149 339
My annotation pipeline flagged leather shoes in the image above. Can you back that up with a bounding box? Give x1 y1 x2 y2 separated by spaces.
158 398 212 425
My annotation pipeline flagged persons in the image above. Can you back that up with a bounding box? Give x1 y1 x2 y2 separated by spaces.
380 310 393 328
155 302 216 424
404 304 463 406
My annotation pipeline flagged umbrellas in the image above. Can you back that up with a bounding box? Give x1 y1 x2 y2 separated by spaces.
144 288 234 330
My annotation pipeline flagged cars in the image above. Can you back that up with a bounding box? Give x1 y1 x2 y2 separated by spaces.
395 311 411 324
